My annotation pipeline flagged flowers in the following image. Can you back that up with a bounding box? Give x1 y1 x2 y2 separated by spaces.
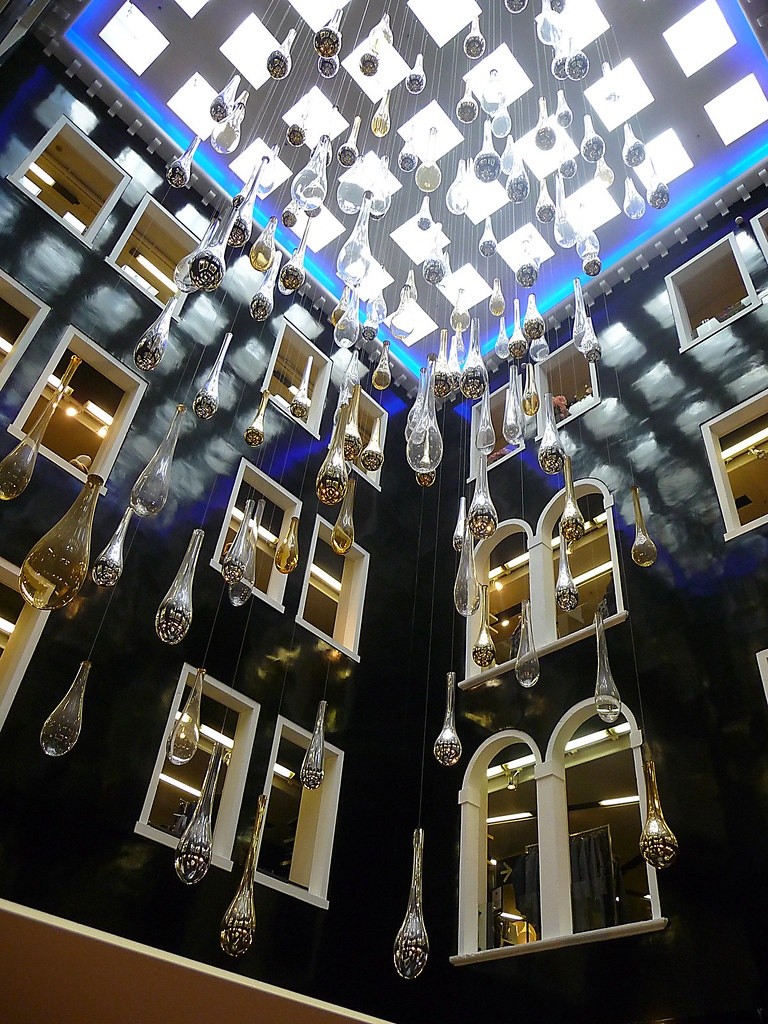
551 395 572 424
488 447 512 469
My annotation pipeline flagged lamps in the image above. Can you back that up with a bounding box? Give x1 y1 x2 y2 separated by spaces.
721 426 768 460
749 446 766 461
0 0 676 982
734 216 744 228
